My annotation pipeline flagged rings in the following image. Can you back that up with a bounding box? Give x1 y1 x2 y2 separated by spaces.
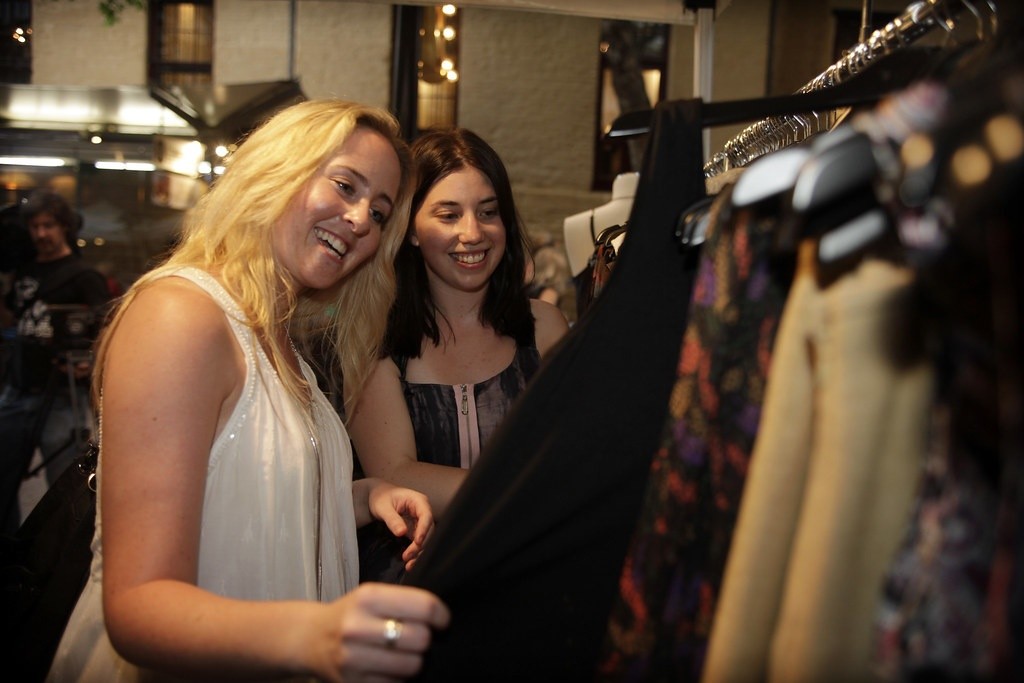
380 617 402 645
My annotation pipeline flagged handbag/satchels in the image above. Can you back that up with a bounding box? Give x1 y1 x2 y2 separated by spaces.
1 440 98 682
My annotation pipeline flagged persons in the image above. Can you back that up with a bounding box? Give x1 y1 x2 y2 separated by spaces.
43 98 452 683
0 179 118 596
564 169 641 325
329 123 570 604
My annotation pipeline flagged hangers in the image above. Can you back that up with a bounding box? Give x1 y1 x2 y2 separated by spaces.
601 0 1000 285
